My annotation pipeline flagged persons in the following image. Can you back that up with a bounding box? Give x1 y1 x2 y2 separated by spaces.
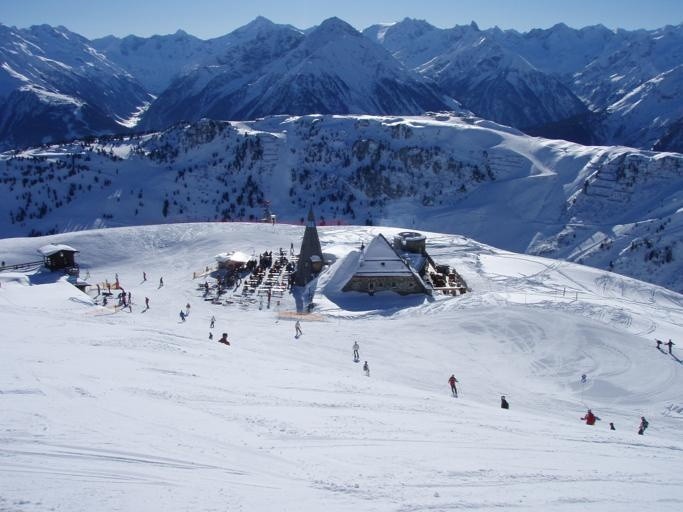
655 339 663 349
501 396 509 409
352 341 359 360
179 304 193 323
664 339 676 353
96 273 166 312
363 361 369 377
205 242 295 308
448 375 459 397
206 315 230 342
295 321 302 337
581 409 600 425
639 417 648 435
